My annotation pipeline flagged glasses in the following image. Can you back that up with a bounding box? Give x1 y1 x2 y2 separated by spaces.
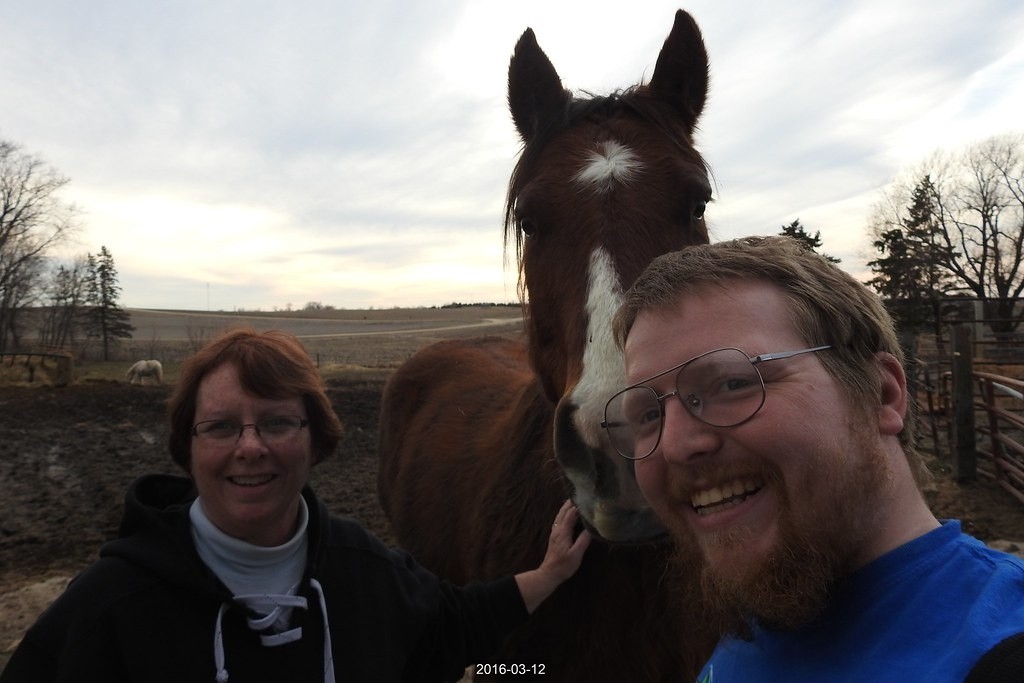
188 414 309 448
601 345 836 460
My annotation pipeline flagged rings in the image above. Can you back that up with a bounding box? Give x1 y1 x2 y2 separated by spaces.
552 524 557 527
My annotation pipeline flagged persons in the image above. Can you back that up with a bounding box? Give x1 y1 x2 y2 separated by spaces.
0 327 593 683
613 236 1024 683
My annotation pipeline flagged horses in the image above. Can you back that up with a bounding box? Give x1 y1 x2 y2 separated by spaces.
378 11 721 683
125 360 163 386
